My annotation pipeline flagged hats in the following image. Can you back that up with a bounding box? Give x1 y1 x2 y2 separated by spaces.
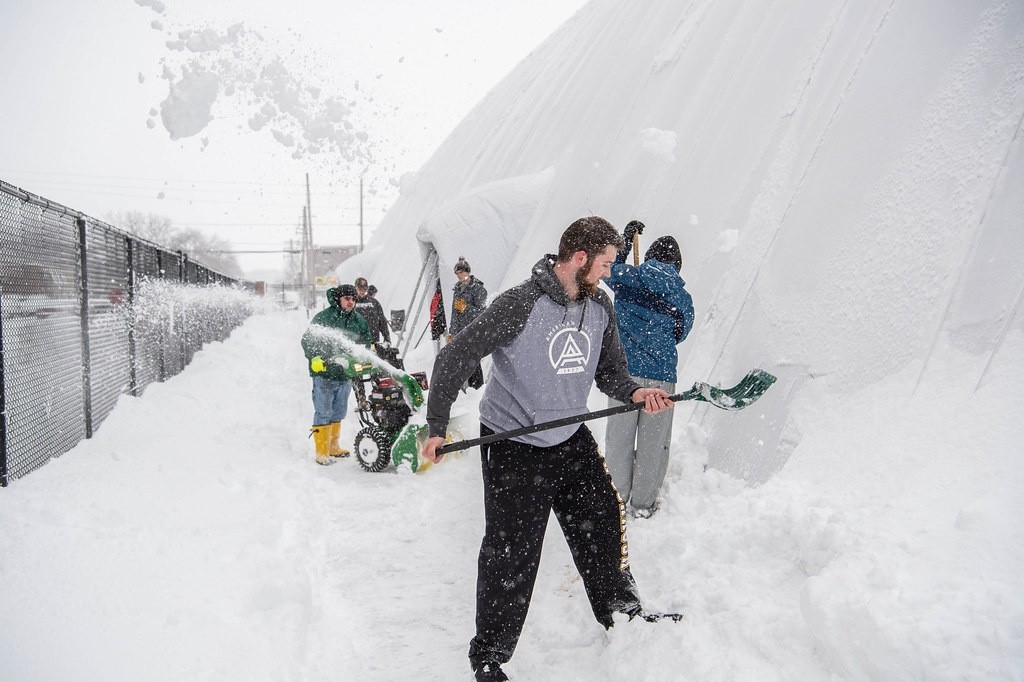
333 284 358 298
644 236 681 273
454 256 470 272
368 285 378 293
355 278 368 289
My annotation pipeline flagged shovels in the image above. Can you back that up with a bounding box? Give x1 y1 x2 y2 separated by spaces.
384 309 406 333
435 367 777 456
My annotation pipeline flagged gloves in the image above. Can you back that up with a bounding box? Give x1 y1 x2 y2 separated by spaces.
311 355 326 373
448 335 453 342
624 220 645 242
454 298 467 312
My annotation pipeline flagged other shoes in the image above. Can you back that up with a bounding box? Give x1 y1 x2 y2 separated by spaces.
629 608 683 624
471 660 509 682
630 501 661 520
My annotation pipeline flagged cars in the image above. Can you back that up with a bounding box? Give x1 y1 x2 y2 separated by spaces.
276 291 301 310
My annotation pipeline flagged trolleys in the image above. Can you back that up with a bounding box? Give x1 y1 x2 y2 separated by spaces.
349 340 429 413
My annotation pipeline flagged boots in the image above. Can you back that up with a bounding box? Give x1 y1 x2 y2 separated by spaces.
309 423 350 466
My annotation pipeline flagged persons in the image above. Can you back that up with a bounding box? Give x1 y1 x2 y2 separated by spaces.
301 283 373 465
351 277 391 414
367 285 377 298
415 216 684 682
601 217 696 518
446 254 488 392
